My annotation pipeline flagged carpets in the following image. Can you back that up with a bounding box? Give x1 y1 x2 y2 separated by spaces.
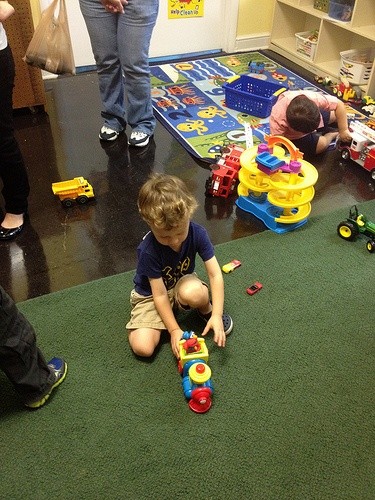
121 49 369 166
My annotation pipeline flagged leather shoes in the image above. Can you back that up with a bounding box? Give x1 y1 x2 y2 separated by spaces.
0 212 24 240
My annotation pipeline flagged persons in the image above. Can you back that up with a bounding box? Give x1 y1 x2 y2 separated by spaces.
79 0 159 147
125 172 234 357
269 89 351 157
0 285 67 410
0 0 31 240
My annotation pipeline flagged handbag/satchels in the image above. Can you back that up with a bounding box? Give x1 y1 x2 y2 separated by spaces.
22 0 76 76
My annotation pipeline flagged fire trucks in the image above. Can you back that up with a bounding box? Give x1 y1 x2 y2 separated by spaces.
204 143 247 204
334 120 375 182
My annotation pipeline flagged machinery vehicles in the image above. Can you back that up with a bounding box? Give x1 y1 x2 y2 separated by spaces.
52 176 97 209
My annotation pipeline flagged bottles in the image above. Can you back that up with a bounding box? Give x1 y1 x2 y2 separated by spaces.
25 51 76 76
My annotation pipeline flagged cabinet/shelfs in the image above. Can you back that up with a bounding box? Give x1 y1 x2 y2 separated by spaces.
267 0 375 99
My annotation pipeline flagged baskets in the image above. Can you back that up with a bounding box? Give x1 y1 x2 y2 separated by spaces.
221 75 288 119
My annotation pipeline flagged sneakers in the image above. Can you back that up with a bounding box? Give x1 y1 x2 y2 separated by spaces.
99 127 119 141
128 131 150 146
23 357 68 408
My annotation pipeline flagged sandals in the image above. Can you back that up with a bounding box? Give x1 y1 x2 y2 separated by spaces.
196 301 233 336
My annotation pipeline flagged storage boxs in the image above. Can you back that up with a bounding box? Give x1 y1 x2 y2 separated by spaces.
222 74 288 119
294 30 318 61
